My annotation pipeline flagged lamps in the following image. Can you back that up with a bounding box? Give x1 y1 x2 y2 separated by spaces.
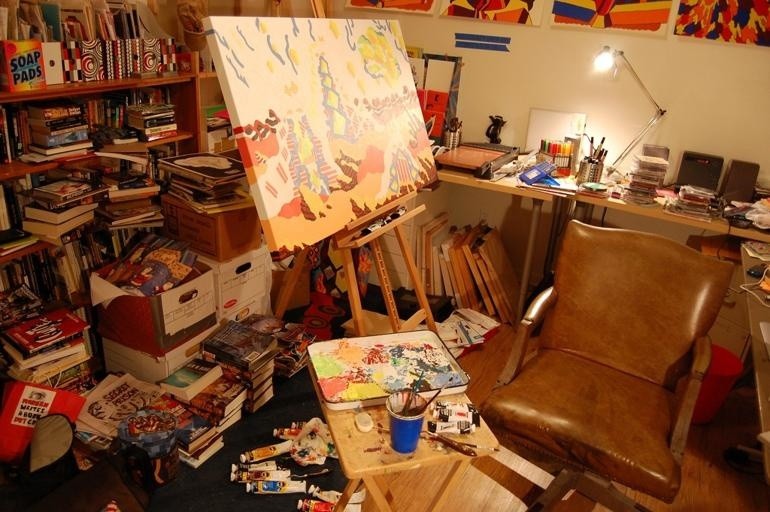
589 43 666 180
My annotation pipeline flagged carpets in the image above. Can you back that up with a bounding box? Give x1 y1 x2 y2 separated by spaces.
120 282 456 512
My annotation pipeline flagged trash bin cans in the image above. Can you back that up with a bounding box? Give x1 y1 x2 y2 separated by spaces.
692 344 744 425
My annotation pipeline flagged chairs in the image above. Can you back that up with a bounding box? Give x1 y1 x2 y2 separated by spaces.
479 220 736 511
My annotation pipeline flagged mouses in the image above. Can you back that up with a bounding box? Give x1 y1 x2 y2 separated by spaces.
747 262 766 278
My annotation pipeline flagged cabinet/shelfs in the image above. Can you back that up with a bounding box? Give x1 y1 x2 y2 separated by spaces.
0 51 239 324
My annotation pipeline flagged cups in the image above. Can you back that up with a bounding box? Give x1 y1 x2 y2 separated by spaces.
385 390 428 456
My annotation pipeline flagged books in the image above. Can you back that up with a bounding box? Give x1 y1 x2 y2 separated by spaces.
1 86 178 163
0 224 202 395
158 152 254 214
71 314 316 469
1 141 178 256
2 1 142 42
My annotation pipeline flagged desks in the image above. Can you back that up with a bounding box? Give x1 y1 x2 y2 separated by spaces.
573 183 770 248
740 245 770 483
306 356 501 512
418 164 554 334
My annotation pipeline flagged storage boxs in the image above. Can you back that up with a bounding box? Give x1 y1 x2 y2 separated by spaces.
90 254 217 352
99 318 216 381
159 194 261 262
182 244 266 324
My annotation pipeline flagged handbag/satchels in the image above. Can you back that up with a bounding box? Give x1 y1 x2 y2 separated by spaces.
0 378 86 466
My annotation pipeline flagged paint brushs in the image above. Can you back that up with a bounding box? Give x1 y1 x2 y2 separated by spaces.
460 443 501 451
403 370 454 417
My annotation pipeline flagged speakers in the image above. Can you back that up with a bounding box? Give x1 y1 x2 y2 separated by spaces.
720 159 759 201
672 152 724 192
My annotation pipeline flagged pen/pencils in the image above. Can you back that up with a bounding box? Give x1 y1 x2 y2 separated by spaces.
584 137 608 165
546 174 560 186
541 138 574 156
443 117 463 133
